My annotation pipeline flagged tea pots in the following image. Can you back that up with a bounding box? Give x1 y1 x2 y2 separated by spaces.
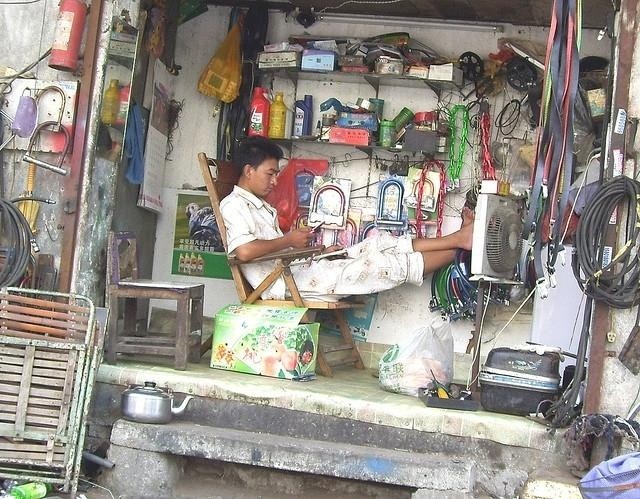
120 379 194 425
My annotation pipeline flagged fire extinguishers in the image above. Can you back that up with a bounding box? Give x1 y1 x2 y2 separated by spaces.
49 0 90 72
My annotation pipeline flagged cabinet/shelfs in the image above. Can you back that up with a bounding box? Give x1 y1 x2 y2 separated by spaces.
251 64 454 154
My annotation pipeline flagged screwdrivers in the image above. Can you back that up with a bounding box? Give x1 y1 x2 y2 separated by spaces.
430 369 450 398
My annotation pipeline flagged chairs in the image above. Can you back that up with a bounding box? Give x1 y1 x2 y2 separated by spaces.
191 150 368 377
0 280 108 499
102 228 206 373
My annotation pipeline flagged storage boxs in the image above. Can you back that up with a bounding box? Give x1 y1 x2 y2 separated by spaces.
259 51 300 70
328 126 372 147
302 49 337 73
404 127 438 153
480 346 564 419
430 61 463 88
339 109 378 130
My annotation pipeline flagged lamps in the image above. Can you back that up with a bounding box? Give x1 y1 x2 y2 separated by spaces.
316 9 508 34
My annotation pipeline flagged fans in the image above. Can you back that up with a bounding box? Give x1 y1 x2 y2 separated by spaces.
463 185 527 285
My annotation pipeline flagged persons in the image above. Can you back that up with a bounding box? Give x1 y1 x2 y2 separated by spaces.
215 132 476 304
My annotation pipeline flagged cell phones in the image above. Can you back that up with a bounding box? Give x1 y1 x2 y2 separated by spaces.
311 221 326 232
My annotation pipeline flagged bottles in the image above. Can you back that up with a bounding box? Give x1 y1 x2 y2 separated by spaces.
99 78 120 126
269 90 287 139
248 86 270 138
293 94 314 137
178 251 206 275
414 113 435 123
368 95 385 120
10 480 53 499
379 107 417 148
